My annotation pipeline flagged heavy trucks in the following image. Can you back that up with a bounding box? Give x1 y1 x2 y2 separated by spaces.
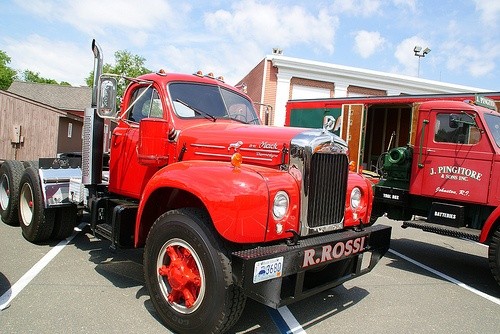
283 91 500 289
0 37 391 333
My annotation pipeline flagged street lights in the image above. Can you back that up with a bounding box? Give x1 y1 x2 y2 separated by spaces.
413 46 431 78
